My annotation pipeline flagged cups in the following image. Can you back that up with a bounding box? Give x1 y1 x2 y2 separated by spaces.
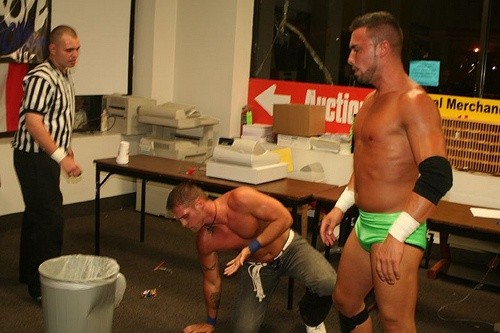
116 141 130 165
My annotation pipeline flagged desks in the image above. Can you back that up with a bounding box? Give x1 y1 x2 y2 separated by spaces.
93 154 338 312
305 183 500 295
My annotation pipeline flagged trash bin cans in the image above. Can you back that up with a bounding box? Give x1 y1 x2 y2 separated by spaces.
39 254 126 333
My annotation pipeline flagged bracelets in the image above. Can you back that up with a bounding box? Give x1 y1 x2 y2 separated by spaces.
50 147 67 163
205 316 217 326
334 186 355 214
248 239 261 254
388 209 421 243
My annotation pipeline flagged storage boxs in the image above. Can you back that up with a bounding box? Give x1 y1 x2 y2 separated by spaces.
272 104 326 136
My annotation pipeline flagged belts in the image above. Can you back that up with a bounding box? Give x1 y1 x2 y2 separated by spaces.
247 228 294 301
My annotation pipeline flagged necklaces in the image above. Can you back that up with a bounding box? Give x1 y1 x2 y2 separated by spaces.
203 200 217 234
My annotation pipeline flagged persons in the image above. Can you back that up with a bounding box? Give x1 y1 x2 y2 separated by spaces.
166 183 337 333
320 11 453 333
10 24 82 306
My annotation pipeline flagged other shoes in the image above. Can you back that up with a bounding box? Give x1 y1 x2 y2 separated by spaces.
305 322 326 333
30 291 41 305
